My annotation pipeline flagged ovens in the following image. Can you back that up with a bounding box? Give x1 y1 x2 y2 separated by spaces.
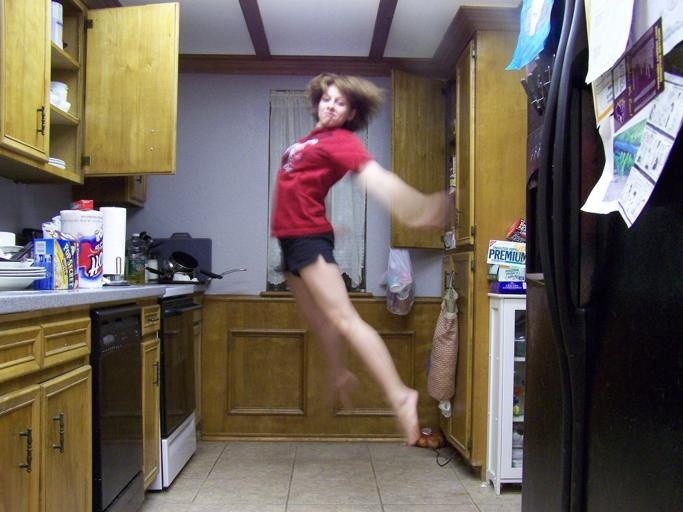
157 294 203 488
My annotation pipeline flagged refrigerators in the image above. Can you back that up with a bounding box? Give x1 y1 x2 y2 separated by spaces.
92 304 145 512
522 0 683 512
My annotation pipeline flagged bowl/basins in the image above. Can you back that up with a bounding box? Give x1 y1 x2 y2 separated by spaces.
50 81 71 112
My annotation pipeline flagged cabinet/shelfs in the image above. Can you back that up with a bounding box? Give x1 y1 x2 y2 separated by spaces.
484 290 527 493
0 303 93 512
139 297 162 492
70 175 148 211
193 294 202 427
390 4 528 494
0 0 180 188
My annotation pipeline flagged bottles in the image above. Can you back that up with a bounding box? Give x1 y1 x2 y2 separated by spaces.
125 234 146 284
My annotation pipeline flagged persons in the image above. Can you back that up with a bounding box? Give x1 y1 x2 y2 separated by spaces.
269 70 453 446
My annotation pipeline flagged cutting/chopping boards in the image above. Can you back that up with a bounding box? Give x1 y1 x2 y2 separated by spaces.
146 238 212 280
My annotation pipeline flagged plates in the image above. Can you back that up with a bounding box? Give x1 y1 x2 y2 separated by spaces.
48 156 67 169
0 245 48 291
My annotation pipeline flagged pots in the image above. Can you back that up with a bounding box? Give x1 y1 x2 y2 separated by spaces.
145 251 247 283
